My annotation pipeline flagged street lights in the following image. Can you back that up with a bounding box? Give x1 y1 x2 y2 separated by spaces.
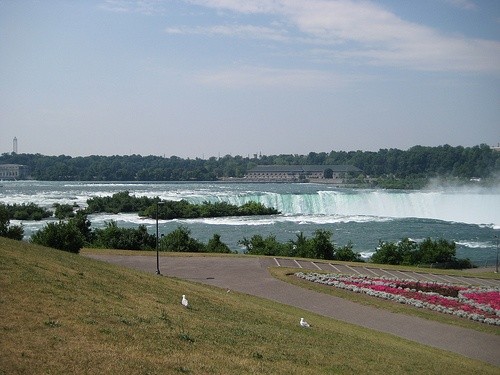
156 202 166 275
